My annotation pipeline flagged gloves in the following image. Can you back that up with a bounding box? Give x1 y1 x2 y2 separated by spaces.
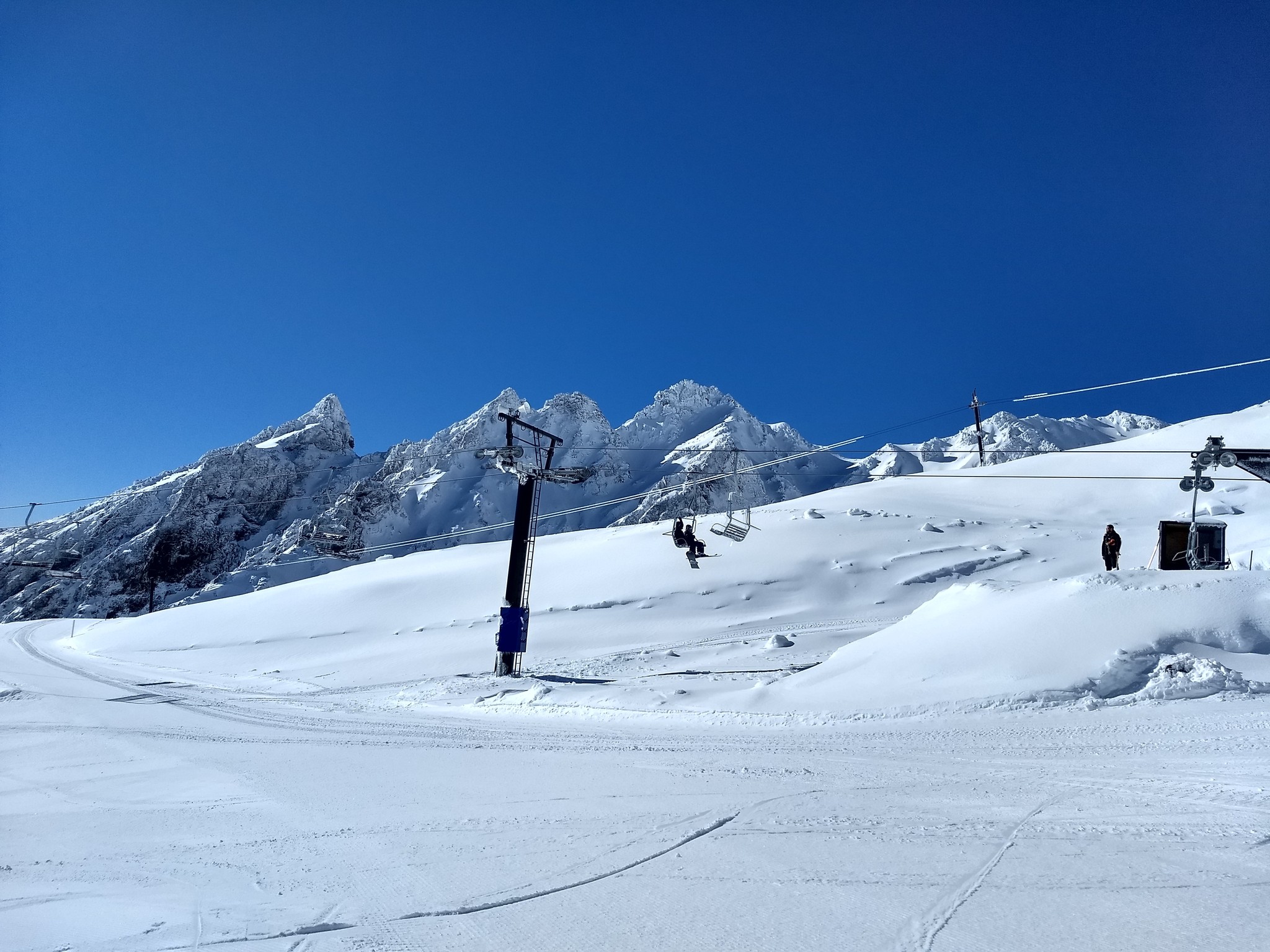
678 517 682 519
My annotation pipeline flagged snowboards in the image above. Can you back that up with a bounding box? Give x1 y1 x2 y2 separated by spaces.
686 551 699 568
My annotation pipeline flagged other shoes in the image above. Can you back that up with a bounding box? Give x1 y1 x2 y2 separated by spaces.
695 555 700 558
700 554 708 557
697 553 700 555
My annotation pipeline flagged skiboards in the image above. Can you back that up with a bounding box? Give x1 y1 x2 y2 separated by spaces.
686 554 722 559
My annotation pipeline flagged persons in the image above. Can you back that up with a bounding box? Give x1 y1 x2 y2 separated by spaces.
675 517 707 556
1102 525 1122 571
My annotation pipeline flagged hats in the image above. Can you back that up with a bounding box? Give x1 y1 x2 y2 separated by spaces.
676 522 681 526
686 525 691 529
